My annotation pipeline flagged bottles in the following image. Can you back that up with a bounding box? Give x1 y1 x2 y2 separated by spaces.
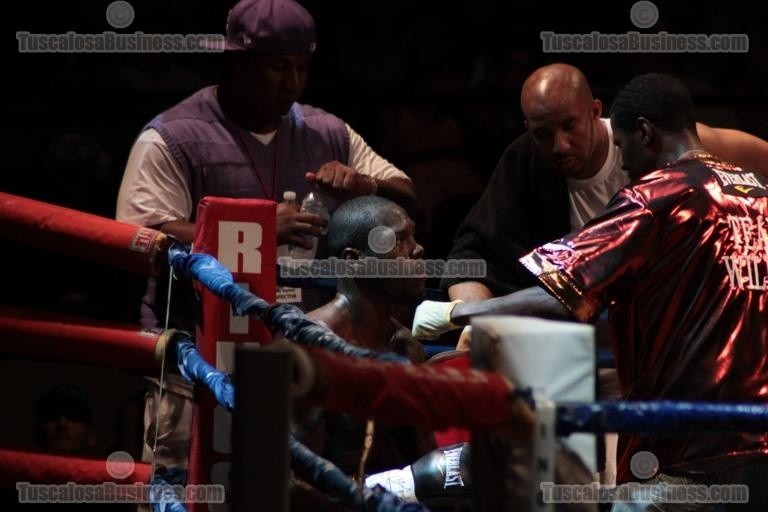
278 181 328 257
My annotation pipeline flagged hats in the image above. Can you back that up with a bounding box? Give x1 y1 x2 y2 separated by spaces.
199 3 318 53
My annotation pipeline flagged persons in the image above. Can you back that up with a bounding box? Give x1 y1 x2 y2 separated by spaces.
33 1 768 511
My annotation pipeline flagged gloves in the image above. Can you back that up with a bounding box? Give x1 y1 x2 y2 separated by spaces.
365 441 473 512
411 299 463 341
